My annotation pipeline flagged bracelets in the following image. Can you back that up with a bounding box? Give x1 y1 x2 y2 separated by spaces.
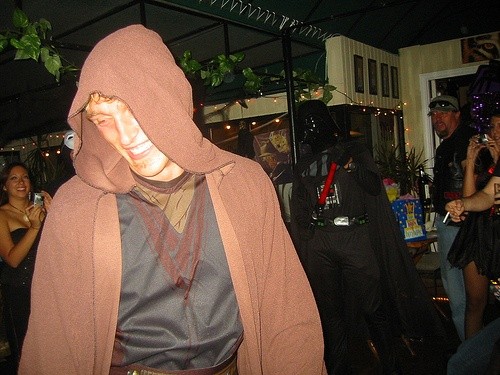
30 226 39 231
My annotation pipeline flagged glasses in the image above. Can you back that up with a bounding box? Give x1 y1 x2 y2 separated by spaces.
429 100 456 108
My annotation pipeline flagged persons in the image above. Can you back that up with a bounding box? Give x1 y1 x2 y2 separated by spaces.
17 24 328 375
0 161 52 375
290 99 444 375
428 91 500 341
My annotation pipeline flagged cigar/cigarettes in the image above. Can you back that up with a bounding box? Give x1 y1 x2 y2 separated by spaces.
443 211 450 223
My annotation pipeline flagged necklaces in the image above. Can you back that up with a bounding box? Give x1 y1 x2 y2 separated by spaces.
8 202 29 222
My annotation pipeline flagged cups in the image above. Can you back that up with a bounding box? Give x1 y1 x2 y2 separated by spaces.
494 182 500 216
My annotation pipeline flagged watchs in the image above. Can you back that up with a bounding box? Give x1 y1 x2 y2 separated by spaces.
344 158 355 173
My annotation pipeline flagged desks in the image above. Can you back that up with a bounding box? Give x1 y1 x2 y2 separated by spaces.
404 236 437 257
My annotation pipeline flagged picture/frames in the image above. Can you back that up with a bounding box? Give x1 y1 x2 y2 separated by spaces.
354 55 364 93
391 66 399 99
381 63 389 97
368 58 377 95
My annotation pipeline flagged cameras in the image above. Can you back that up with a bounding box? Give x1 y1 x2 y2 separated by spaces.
34 193 43 207
476 134 488 144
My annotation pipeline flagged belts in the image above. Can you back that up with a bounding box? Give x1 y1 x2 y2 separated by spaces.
310 213 370 227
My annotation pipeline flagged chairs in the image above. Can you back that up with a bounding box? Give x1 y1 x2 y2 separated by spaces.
415 239 442 305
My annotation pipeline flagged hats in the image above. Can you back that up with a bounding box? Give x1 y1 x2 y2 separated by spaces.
429 95 465 119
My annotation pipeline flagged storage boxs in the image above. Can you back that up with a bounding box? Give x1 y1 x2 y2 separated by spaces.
390 199 426 241
